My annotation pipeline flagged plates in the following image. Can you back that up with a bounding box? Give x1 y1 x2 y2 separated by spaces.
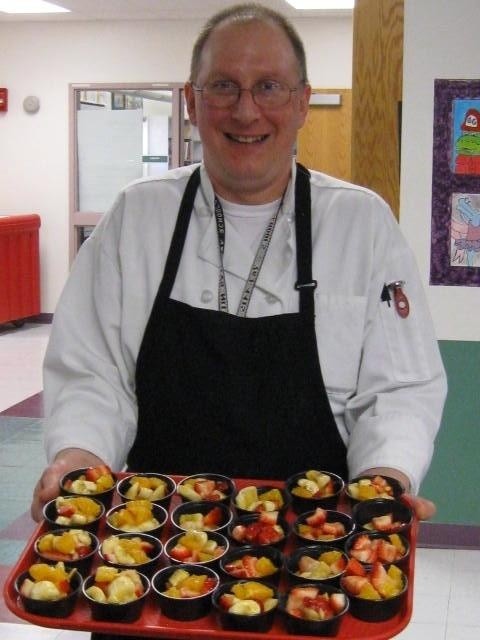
3 472 416 640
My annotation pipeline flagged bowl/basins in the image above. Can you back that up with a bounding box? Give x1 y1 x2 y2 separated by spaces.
34 528 99 574
170 503 233 536
279 584 349 636
98 533 163 568
118 473 176 512
228 514 291 547
346 530 410 565
42 495 105 536
292 510 356 549
345 474 405 503
81 571 150 623
211 579 281 633
232 486 290 523
107 502 169 539
59 466 117 509
14 566 83 619
164 530 229 565
352 498 414 531
150 564 219 622
286 545 351 581
219 544 285 582
284 470 345 514
176 473 235 503
341 565 408 622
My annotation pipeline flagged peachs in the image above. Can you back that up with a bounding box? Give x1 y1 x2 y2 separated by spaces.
318 551 342 565
38 534 76 555
125 476 167 501
255 557 277 576
107 500 152 528
162 569 207 598
231 581 274 600
355 486 375 500
117 537 149 564
177 535 211 562
96 473 114 492
387 534 402 550
59 496 100 524
28 564 72 597
293 524 335 541
359 564 403 600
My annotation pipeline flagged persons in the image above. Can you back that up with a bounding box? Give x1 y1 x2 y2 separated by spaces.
30 5 447 524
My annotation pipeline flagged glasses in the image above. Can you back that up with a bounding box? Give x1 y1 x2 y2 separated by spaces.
187 76 312 108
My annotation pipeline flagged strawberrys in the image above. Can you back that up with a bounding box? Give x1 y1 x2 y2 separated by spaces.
313 479 334 499
202 506 222 526
220 593 240 613
58 580 70 593
349 534 397 565
200 577 218 595
253 599 264 616
85 465 111 481
193 479 228 501
254 504 267 511
305 508 346 541
283 587 345 620
95 581 110 592
372 513 403 534
135 589 145 597
170 545 193 561
232 511 284 544
340 558 387 595
75 546 90 559
140 541 154 554
225 554 262 580
297 556 321 571
336 559 347 571
56 506 75 517
